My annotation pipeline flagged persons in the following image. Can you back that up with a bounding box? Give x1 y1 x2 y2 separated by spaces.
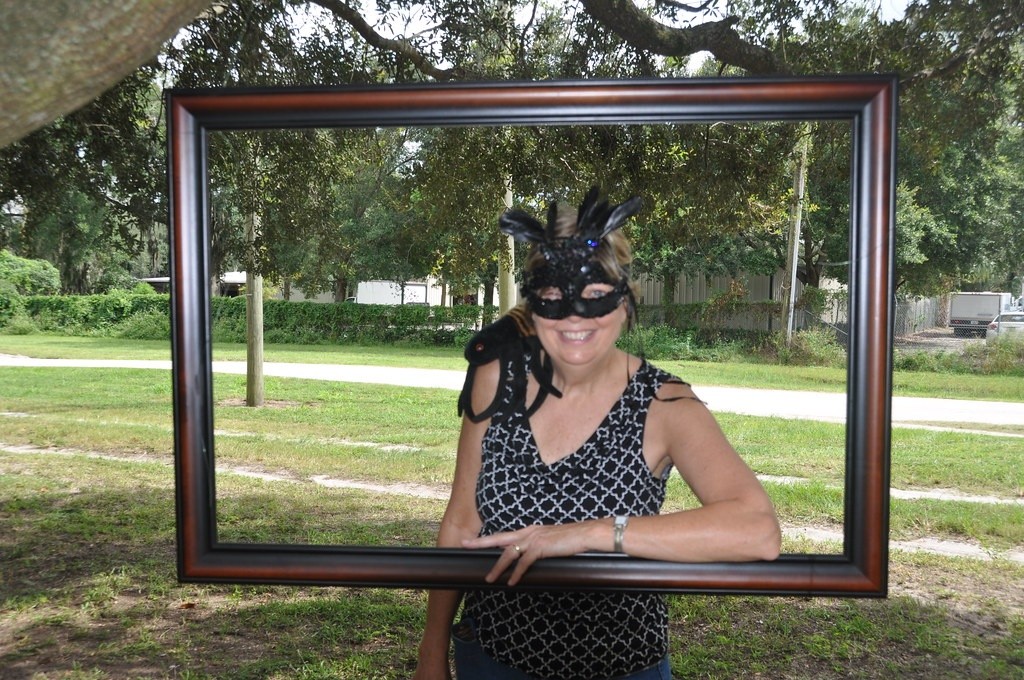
416 188 781 680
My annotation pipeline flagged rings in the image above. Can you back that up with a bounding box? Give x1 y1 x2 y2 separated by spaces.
513 544 523 555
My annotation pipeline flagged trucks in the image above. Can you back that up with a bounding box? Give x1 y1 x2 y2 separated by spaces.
948 292 1011 338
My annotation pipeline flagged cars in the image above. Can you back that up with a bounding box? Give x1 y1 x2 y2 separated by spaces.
984 312 1024 345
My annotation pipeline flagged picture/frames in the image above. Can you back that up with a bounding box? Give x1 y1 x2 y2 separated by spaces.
164 72 901 600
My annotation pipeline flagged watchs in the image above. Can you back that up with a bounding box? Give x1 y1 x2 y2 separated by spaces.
614 515 628 552
